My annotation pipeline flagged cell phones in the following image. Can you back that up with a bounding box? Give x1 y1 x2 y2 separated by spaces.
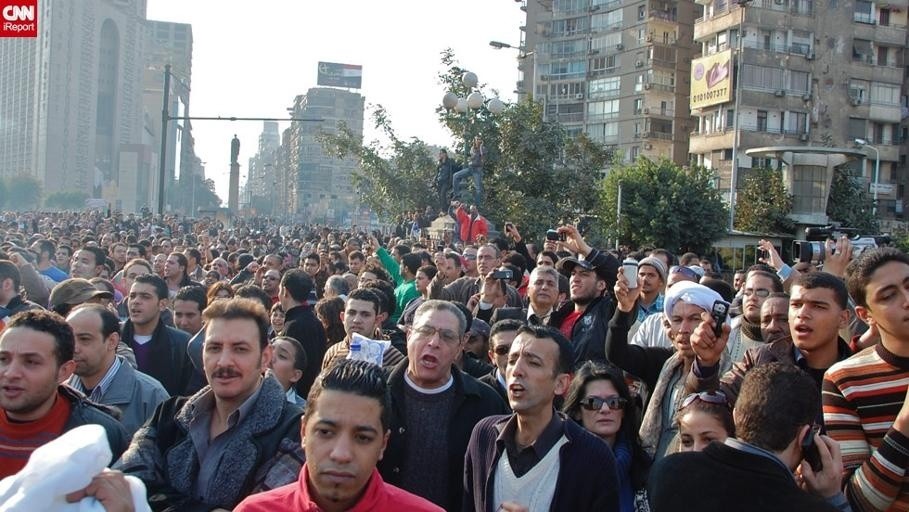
801 424 827 473
437 245 443 251
506 224 512 232
623 259 639 289
711 300 730 338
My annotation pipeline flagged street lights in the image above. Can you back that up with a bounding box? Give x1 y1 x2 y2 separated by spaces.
490 41 538 104
614 177 628 250
442 72 505 202
729 0 752 230
855 137 881 219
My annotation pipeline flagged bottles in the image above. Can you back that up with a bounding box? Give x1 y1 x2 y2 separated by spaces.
346 343 365 363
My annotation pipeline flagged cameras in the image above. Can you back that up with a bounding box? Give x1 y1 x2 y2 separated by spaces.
546 230 566 241
493 270 513 279
792 226 890 264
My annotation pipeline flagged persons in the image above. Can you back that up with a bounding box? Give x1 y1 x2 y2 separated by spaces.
451 135 488 207
432 149 457 217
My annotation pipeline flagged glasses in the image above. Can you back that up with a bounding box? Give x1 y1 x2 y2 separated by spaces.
677 389 733 413
411 323 460 344
744 289 773 299
669 265 696 280
579 396 627 411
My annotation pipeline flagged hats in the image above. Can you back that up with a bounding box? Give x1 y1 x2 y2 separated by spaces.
562 258 595 273
637 256 667 283
468 317 492 339
46 277 114 311
687 265 706 278
663 279 731 328
